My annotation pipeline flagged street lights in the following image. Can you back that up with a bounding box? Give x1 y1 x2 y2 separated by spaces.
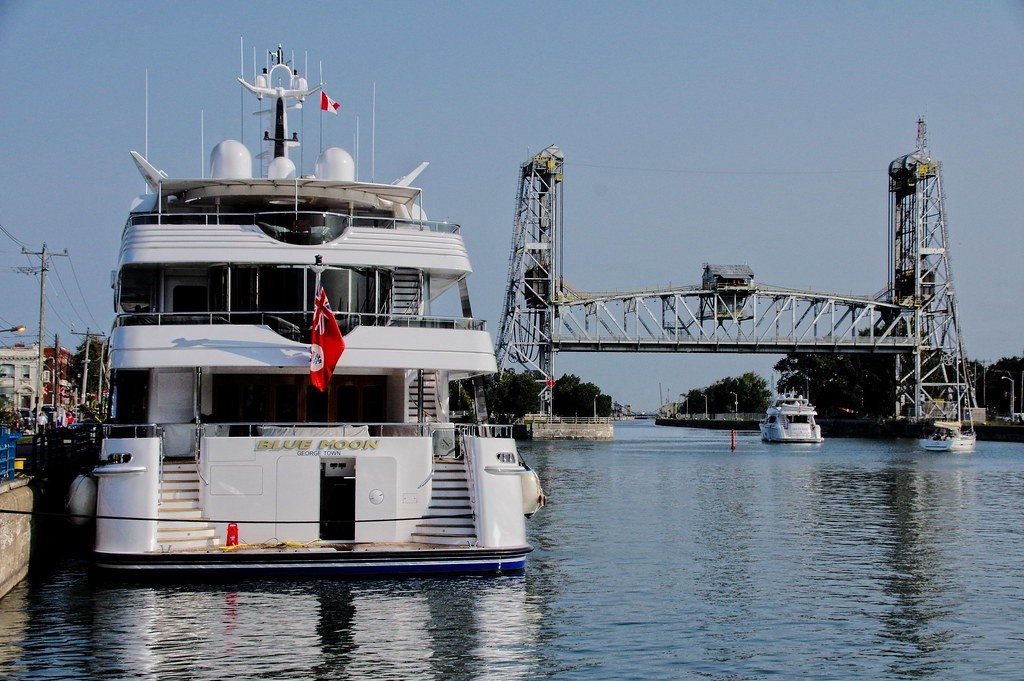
701 394 707 420
685 397 688 415
730 392 737 420
1001 376 1015 422
594 394 598 421
0 325 26 333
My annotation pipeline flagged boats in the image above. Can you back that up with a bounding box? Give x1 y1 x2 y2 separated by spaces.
758 387 824 444
919 421 977 451
90 33 546 580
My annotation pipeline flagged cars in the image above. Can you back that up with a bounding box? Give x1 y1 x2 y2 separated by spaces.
17 408 34 426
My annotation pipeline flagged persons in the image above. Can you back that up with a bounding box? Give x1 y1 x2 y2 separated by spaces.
37 413 48 433
933 433 948 441
1018 414 1022 422
66 412 74 427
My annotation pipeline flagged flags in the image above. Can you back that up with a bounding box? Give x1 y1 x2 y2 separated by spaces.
322 92 339 115
309 287 345 392
